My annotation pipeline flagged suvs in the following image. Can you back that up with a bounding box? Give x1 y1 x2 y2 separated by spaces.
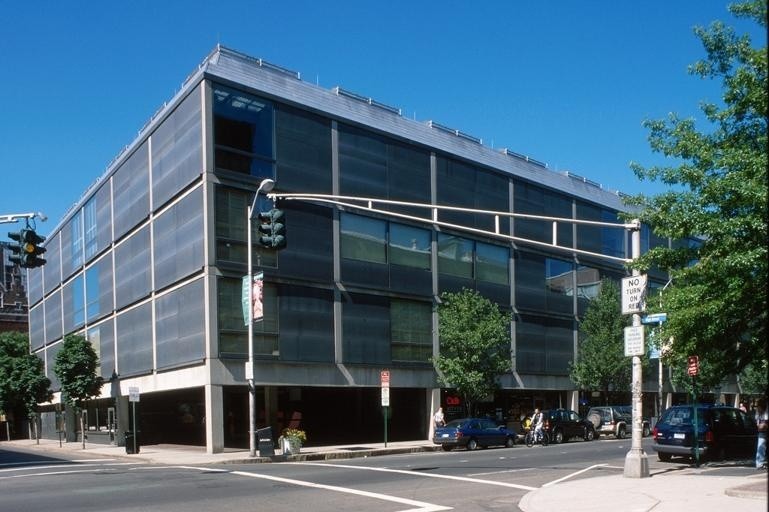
584 405 650 438
652 404 759 461
523 408 596 442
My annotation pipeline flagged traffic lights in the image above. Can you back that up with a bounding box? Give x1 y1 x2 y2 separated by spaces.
258 210 273 249
18 230 36 268
34 234 47 268
6 230 22 264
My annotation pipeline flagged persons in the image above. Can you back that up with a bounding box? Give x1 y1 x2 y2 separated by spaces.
755 388 769 469
433 408 447 429
739 403 746 413
529 408 544 443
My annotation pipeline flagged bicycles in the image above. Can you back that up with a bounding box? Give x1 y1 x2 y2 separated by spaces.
525 427 549 448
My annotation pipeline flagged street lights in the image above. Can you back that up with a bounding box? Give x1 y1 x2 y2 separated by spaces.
244 179 278 458
658 272 688 417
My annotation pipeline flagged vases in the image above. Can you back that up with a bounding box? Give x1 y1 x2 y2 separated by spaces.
281 437 300 455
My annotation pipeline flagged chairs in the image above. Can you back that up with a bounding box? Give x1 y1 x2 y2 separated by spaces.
671 414 683 423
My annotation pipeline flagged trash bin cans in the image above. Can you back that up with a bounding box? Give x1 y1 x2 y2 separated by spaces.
125 430 141 454
257 426 274 457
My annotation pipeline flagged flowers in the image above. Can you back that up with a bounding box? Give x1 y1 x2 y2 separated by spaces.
283 428 307 449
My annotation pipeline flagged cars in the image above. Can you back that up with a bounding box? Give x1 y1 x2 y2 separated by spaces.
433 417 517 450
